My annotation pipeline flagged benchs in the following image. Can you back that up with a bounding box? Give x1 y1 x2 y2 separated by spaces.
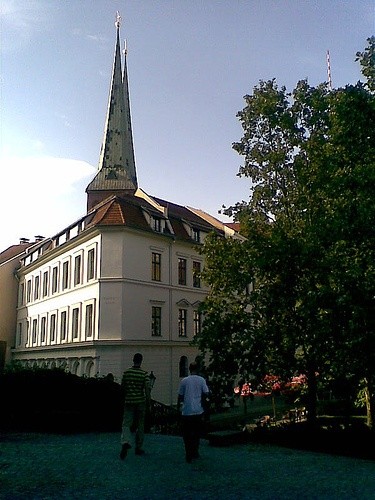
206 430 245 448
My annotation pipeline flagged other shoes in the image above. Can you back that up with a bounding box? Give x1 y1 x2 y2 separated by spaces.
119 445 130 461
135 448 144 455
186 454 201 464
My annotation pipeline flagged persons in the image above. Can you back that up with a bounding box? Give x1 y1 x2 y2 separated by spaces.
120 351 151 461
177 362 210 464
